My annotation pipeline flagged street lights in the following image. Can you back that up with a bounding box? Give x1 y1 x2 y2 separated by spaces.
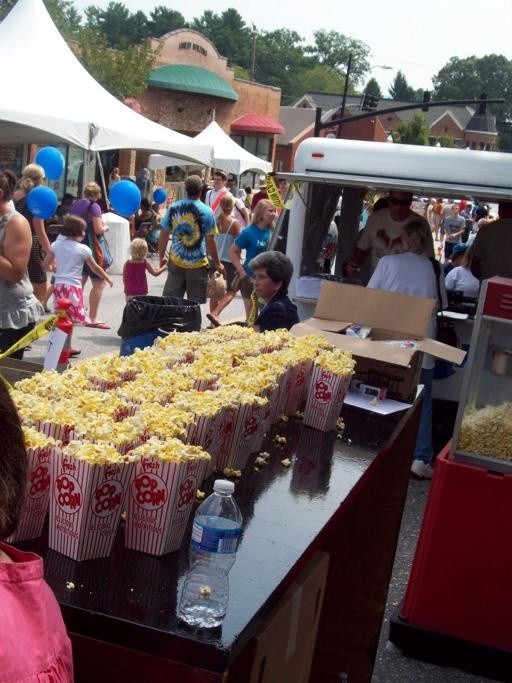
336 53 395 137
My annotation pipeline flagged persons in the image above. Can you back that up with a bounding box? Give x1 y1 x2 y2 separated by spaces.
0 378 75 682
365 220 449 478
317 189 511 306
0 162 300 360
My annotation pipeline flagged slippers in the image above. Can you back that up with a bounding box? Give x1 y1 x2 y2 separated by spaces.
86 322 109 329
206 314 221 329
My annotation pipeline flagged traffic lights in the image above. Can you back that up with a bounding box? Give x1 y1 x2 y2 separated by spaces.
369 96 379 111
361 94 372 112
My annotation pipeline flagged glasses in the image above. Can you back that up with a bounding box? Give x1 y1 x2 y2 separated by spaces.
388 195 411 207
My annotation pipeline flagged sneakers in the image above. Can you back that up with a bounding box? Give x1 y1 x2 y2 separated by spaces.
69 348 80 356
412 459 433 477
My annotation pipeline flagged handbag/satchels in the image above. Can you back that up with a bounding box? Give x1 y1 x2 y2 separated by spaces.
230 270 241 292
87 231 113 277
434 322 461 379
205 270 220 298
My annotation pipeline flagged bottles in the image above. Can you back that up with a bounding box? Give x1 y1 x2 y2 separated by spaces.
322 256 330 274
177 479 244 631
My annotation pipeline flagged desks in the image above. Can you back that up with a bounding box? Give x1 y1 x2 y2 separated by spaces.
13 382 422 683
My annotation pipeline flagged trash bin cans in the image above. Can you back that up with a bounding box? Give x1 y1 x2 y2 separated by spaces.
120 295 202 357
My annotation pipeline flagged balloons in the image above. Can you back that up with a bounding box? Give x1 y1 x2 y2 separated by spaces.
34 144 66 180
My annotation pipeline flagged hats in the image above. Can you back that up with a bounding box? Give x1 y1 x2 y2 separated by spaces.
453 244 469 251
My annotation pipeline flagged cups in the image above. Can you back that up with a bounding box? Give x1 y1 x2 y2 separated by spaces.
242 274 249 291
492 347 512 376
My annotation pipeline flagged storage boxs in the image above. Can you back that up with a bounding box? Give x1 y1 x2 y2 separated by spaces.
291 279 471 406
